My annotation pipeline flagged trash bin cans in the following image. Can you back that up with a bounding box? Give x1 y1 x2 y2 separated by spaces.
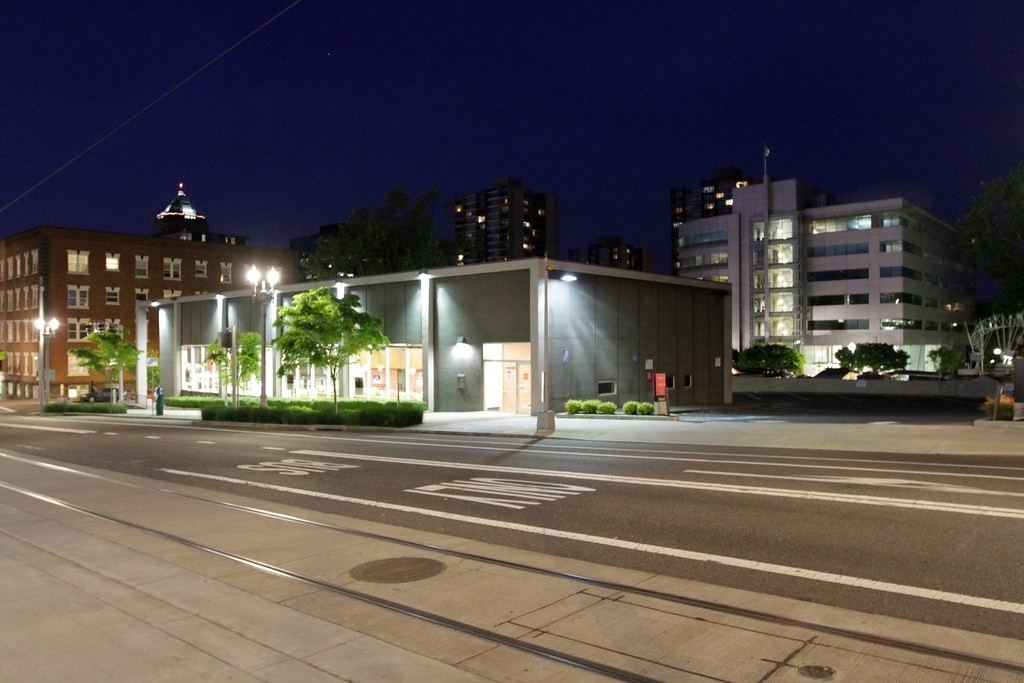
654 401 667 415
537 411 555 431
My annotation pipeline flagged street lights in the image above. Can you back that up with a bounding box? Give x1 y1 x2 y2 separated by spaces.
244 264 279 408
33 315 60 413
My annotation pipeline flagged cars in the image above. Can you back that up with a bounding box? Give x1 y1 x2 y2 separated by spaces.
127 385 157 400
79 388 119 403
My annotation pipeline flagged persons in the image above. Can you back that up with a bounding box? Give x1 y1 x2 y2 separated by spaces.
91 380 97 393
